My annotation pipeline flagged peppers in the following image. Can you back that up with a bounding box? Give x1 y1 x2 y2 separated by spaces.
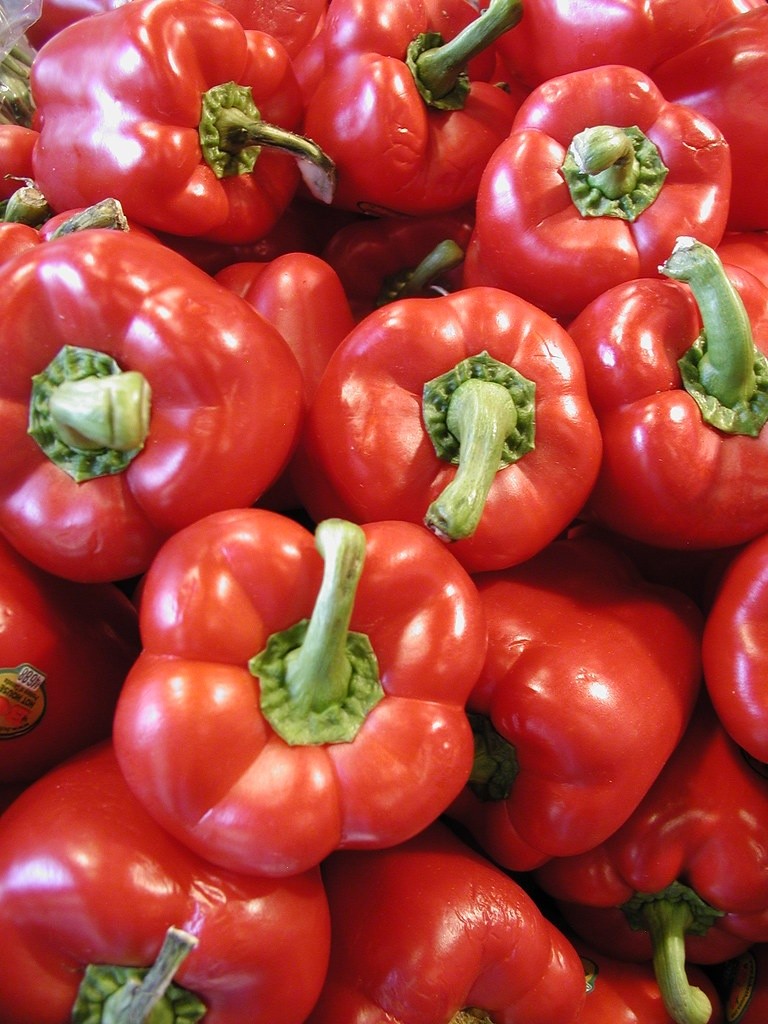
0 0 768 1024
